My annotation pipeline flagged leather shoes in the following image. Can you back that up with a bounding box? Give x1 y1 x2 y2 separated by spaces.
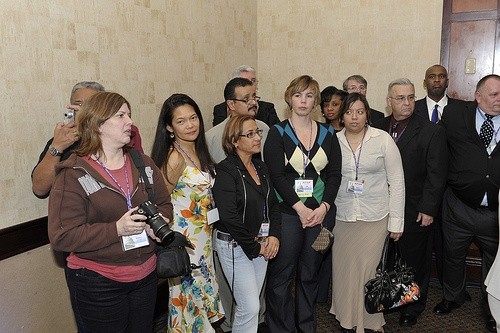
483 292 497 331
434 297 465 315
399 300 426 326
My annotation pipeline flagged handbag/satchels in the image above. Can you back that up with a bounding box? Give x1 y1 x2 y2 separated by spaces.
365 232 423 314
155 232 195 279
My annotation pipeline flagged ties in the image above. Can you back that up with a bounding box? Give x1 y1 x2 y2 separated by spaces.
479 114 494 148
431 104 439 125
390 122 399 141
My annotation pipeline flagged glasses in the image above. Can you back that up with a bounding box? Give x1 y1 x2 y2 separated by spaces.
347 86 367 91
248 78 258 85
239 129 264 138
232 95 261 104
389 95 417 102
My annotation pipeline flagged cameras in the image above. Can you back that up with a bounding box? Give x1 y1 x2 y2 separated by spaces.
63 107 75 126
130 200 175 247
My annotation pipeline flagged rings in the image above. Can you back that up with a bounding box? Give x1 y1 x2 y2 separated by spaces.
73 131 79 136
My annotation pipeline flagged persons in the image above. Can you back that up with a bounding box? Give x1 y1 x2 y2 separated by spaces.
374 64 500 333
151 93 226 333
205 65 405 333
49 92 173 333
30 81 106 199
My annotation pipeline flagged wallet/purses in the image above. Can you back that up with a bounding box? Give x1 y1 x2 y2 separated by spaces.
312 227 334 255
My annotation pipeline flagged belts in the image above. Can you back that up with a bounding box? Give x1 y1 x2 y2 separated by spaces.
216 232 236 241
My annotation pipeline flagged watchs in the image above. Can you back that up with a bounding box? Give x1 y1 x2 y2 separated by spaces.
49 145 63 156
